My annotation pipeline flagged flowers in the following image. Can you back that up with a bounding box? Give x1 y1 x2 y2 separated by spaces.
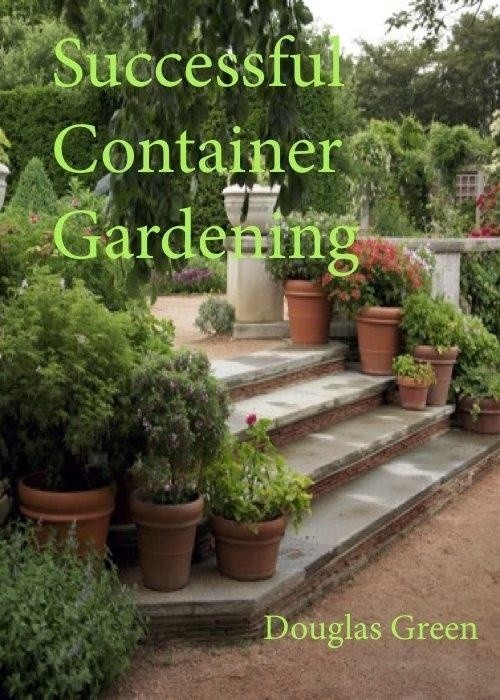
0 158 500 535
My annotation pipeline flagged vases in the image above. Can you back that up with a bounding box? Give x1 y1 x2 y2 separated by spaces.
20 474 289 591
